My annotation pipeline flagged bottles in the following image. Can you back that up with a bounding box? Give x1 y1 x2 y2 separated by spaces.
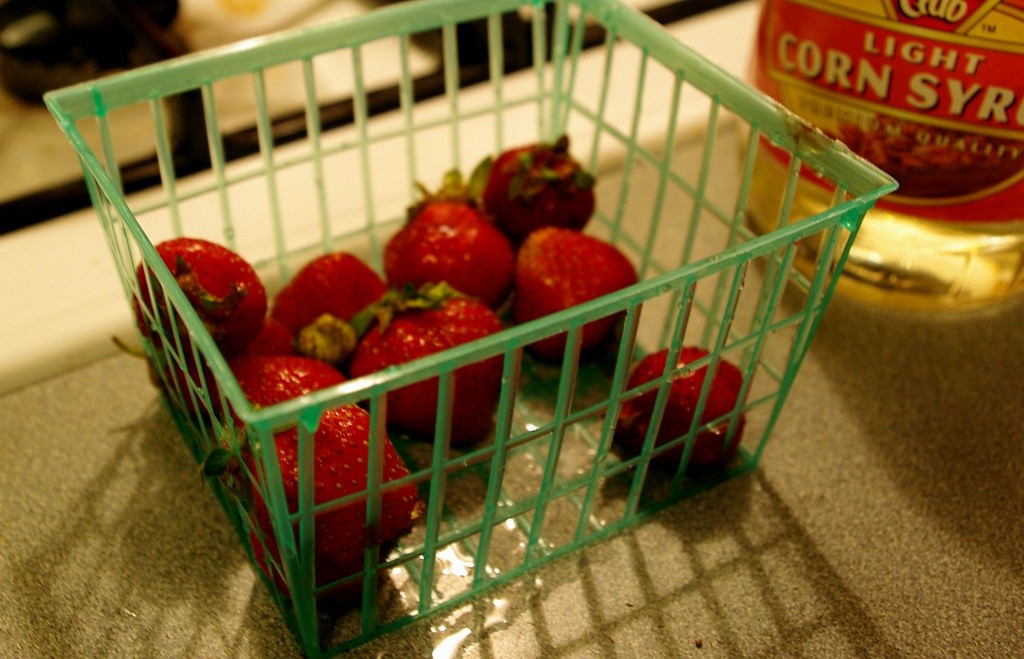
734 0 1024 325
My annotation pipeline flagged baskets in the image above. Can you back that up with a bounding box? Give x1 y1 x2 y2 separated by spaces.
41 0 899 658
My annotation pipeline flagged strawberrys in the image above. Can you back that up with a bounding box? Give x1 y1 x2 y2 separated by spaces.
608 346 747 473
126 129 640 597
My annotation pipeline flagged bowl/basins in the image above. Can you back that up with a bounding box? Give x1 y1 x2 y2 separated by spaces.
0 0 179 104
